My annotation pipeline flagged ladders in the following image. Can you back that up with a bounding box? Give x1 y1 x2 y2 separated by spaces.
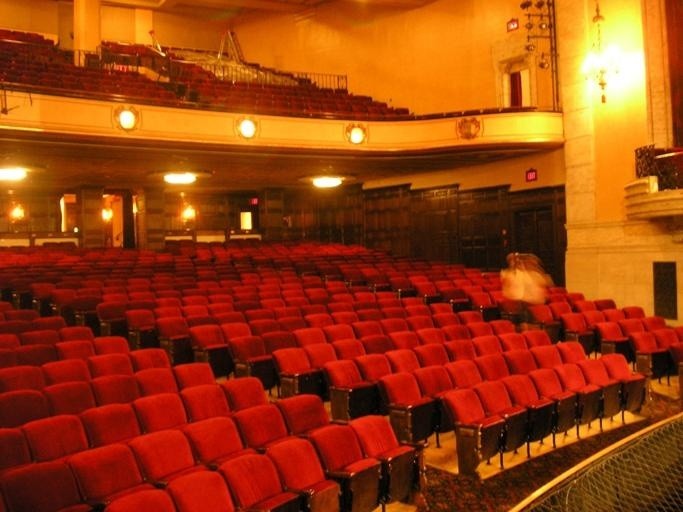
217 30 240 64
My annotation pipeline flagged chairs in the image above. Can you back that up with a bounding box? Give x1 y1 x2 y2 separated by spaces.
1 232 682 512
423 105 537 116
1 27 414 116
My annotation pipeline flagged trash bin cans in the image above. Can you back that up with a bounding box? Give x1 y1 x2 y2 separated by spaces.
449 245 486 268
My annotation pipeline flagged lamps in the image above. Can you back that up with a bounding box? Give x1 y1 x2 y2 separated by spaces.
519 0 556 71
